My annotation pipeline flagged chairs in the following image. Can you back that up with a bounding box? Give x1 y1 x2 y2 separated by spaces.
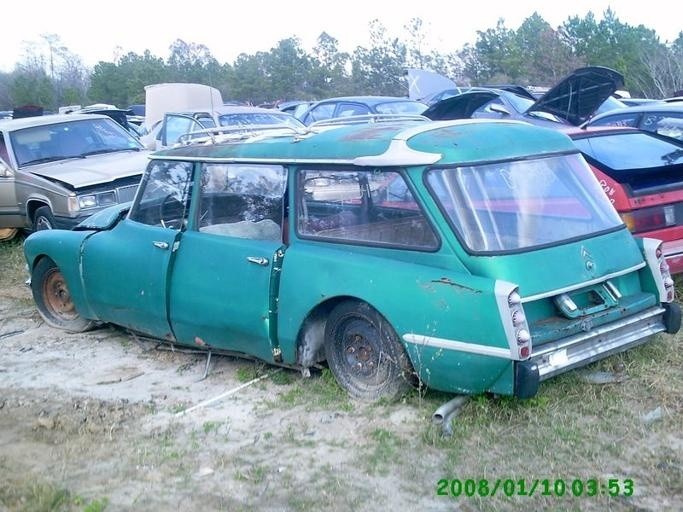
200 210 358 240
